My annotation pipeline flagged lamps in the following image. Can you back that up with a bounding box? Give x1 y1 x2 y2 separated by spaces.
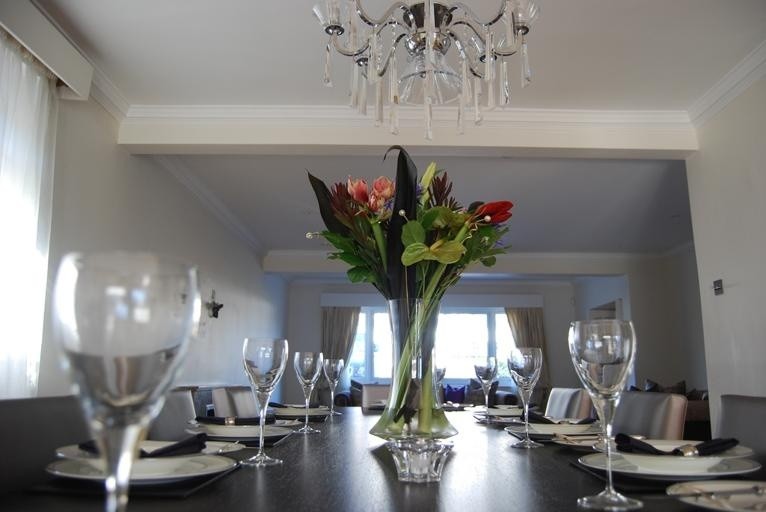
207 289 224 319
310 0 541 144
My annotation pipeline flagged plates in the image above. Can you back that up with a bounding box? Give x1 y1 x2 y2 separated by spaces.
503 424 609 443
665 479 765 512
273 405 331 420
43 454 239 490
187 424 296 444
55 437 247 459
576 452 762 482
551 437 752 455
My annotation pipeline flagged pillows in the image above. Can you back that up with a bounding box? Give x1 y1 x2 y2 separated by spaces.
445 384 465 403
470 378 499 406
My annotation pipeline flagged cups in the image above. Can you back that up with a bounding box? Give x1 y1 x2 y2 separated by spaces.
567 317 646 508
383 441 454 486
433 367 447 410
321 358 345 417
50 249 203 508
293 351 326 436
507 346 544 449
238 337 290 468
473 356 499 417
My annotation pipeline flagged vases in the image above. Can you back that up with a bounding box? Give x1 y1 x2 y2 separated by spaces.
369 299 460 439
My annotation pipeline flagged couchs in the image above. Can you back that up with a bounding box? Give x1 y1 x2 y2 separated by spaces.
628 381 712 443
335 383 518 409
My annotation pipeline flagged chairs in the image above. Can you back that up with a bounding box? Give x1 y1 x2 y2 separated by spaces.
543 387 595 425
211 384 255 418
147 387 199 443
719 392 766 478
611 390 687 441
359 384 420 411
1 395 96 505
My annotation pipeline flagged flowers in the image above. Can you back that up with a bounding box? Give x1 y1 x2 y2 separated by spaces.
305 145 515 440
